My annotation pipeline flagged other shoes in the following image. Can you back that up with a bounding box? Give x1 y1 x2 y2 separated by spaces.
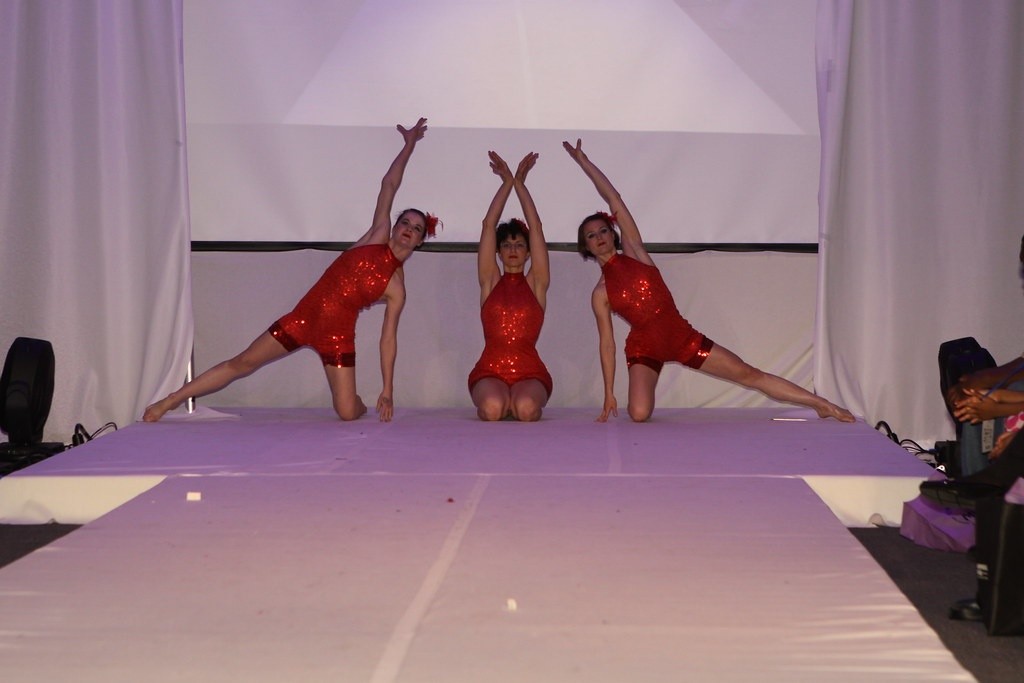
919 477 989 510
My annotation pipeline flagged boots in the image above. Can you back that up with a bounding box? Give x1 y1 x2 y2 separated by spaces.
949 563 989 622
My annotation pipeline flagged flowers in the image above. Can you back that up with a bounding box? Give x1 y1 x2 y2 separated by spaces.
516 218 530 233
427 213 444 239
598 210 618 226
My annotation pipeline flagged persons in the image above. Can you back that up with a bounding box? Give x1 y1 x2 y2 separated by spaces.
142 118 439 422
468 151 553 421
562 138 856 423
948 234 1024 620
